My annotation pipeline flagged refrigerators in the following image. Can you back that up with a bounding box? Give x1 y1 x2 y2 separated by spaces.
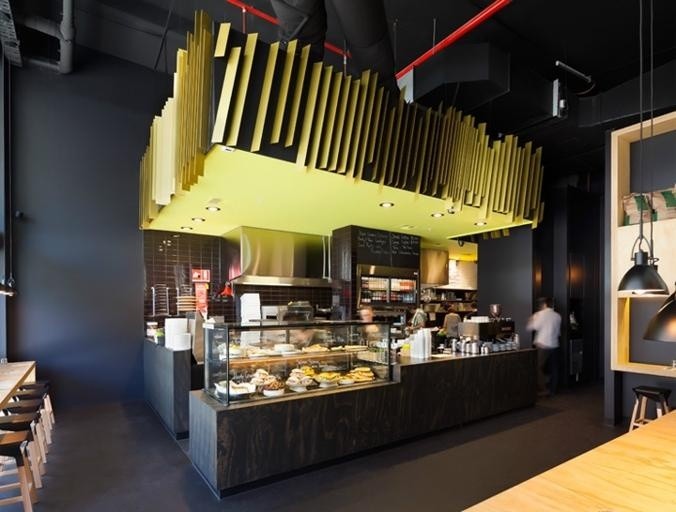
356 263 420 308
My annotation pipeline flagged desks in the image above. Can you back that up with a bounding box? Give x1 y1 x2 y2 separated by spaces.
0 361 37 414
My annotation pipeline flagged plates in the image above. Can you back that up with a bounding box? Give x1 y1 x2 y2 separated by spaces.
219 350 306 360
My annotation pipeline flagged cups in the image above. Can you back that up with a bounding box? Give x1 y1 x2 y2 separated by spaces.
488 304 502 318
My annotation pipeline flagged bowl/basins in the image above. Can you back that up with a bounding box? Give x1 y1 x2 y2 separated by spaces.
274 343 296 352
262 388 285 397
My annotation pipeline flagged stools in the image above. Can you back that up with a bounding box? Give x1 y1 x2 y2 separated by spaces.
623 386 672 434
0 380 56 511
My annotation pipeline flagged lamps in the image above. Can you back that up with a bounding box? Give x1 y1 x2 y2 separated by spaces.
617 1 676 297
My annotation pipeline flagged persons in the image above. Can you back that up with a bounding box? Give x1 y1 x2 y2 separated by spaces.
466 303 478 320
404 303 428 334
525 296 565 400
442 305 463 347
355 305 385 338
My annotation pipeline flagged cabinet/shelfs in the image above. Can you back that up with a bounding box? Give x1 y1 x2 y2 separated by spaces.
202 321 390 407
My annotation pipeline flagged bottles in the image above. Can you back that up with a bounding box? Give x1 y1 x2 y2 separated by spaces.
449 334 489 356
146 321 158 338
